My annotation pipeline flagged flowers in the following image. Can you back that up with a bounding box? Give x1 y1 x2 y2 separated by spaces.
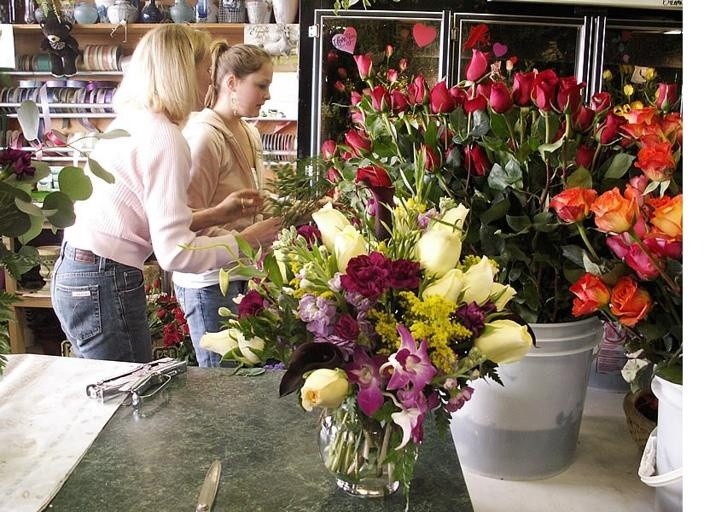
0 101 131 379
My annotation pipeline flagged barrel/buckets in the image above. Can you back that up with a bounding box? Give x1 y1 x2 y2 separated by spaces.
635 370 683 491
449 316 606 483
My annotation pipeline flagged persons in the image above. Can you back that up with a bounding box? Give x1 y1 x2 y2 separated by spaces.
47 23 285 363
166 38 275 368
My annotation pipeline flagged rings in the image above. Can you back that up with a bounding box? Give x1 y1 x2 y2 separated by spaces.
240 197 244 205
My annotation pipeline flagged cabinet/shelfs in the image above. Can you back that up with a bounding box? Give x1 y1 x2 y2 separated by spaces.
0 0 300 354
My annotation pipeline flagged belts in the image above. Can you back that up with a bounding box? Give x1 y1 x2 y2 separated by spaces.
60 243 108 264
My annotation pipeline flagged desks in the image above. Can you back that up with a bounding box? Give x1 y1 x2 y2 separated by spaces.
0 354 666 511
